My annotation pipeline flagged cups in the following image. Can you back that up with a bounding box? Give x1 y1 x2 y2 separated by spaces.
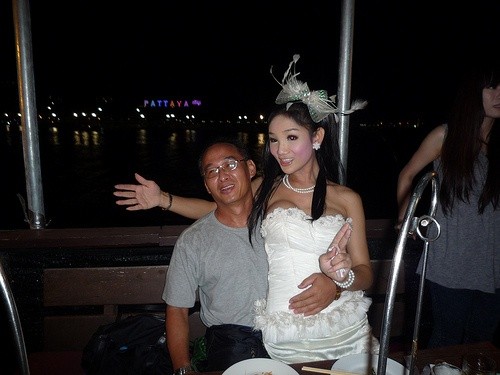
460 351 498 375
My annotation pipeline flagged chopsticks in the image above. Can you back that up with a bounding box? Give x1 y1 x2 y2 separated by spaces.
301 365 360 375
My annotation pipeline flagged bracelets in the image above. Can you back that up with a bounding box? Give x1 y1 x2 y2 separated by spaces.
161 191 173 211
173 367 195 375
335 283 343 300
332 269 355 288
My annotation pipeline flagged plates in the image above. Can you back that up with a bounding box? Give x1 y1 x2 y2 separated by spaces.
221 358 300 375
330 353 406 375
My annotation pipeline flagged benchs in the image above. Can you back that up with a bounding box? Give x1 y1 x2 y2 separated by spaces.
17 257 406 375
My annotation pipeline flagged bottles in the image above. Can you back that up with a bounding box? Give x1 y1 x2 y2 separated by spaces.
173 367 194 375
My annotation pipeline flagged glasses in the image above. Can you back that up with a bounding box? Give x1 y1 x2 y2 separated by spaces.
203 159 248 179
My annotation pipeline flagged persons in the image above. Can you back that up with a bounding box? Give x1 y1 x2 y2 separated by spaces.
394 63 500 350
163 140 345 375
113 100 381 366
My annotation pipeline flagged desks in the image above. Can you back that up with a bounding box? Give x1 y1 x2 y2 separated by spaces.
203 341 500 375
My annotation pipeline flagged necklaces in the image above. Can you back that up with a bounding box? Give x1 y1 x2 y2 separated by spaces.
482 140 487 146
283 174 315 194
